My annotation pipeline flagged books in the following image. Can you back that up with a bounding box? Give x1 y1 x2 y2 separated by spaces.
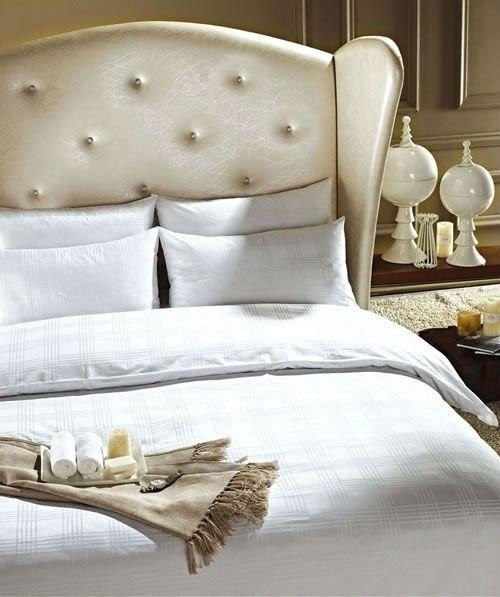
475 351 500 356
457 331 500 353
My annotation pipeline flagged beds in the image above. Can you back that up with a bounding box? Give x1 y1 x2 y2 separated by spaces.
0 17 500 597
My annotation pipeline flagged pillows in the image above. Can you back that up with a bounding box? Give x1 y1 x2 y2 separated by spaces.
0 193 157 250
158 215 361 312
2 226 161 327
155 176 335 238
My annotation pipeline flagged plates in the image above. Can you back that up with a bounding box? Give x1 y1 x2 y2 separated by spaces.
40 438 148 486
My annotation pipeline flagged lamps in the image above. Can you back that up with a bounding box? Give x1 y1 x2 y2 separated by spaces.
380 111 498 269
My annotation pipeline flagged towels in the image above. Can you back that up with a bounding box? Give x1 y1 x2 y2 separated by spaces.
47 429 104 480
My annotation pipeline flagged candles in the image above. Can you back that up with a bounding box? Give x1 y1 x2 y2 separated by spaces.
456 296 500 338
